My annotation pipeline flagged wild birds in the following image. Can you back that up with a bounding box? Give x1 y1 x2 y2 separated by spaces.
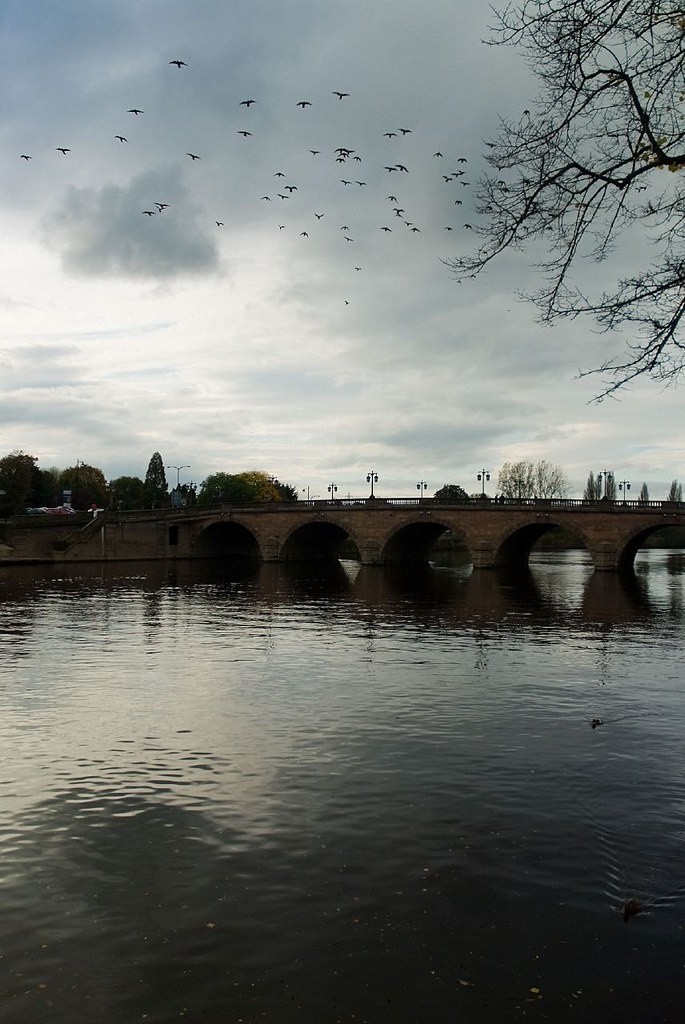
186 154 200 160
297 101 310 109
127 108 144 115
345 301 350 306
20 155 31 161
277 193 288 200
333 91 350 99
260 197 271 202
154 202 169 212
238 130 251 137
274 172 285 178
239 99 256 107
57 147 70 155
285 186 297 192
300 108 586 285
215 221 223 226
278 224 285 230
114 136 127 143
142 211 154 217
169 60 186 69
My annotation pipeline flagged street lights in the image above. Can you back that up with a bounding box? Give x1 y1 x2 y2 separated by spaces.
328 482 337 501
416 480 427 498
76 458 86 510
267 476 279 501
514 478 526 498
599 468 613 496
618 478 631 500
302 486 309 500
477 468 490 493
366 471 378 499
167 465 191 486
